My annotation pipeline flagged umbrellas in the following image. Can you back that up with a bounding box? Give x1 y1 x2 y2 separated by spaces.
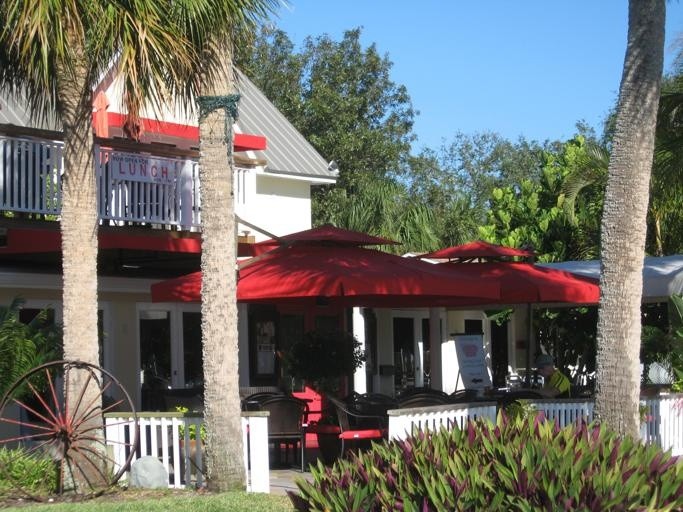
407 236 601 362
150 218 506 382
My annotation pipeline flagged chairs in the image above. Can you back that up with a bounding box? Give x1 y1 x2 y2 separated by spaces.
244 374 397 474
397 374 544 409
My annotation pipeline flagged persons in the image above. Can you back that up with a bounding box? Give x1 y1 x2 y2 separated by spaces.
508 353 571 401
